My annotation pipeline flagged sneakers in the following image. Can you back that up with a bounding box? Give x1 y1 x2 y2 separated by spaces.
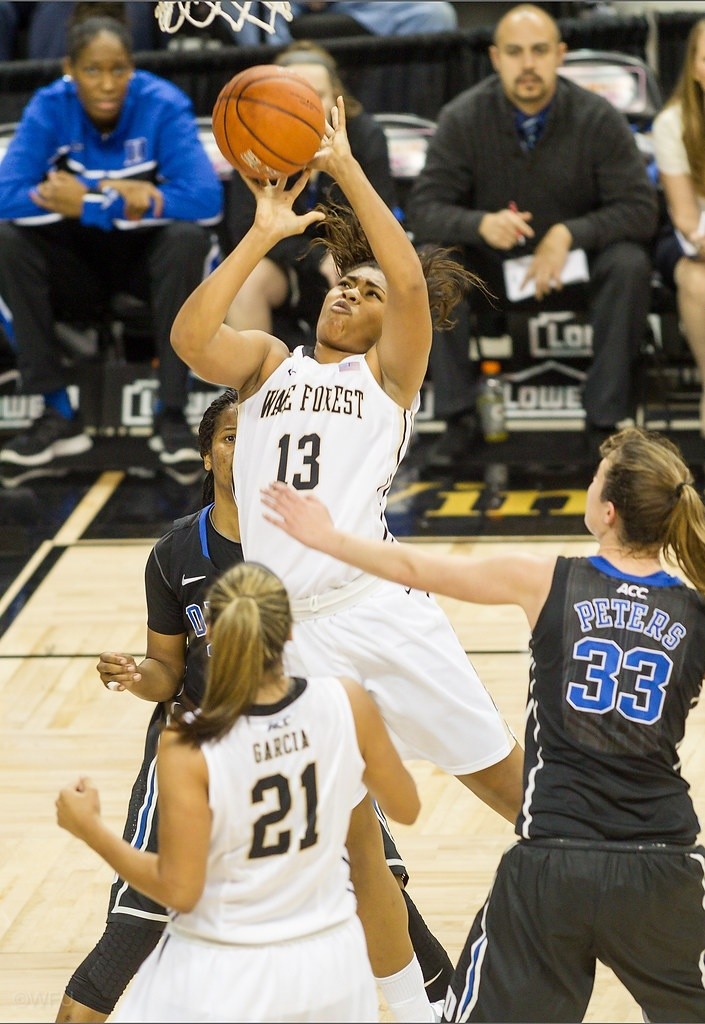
148 420 203 465
0 410 94 467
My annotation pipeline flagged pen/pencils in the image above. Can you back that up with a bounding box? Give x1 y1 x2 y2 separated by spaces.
509 200 527 248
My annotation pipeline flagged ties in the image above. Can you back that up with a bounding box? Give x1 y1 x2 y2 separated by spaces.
518 116 539 151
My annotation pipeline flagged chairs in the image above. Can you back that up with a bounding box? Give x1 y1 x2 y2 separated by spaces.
0 51 662 489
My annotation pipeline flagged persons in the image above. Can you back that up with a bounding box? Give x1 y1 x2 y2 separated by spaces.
258 426 705 1024
23 1 169 62
651 12 705 437
171 93 523 827
220 0 457 50
403 3 660 467
0 16 224 469
209 40 394 330
54 387 455 1022
54 561 423 1023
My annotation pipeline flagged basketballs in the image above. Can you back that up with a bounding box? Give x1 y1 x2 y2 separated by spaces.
210 63 327 180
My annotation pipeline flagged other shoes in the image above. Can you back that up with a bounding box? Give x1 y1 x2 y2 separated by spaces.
579 418 616 473
427 410 484 476
